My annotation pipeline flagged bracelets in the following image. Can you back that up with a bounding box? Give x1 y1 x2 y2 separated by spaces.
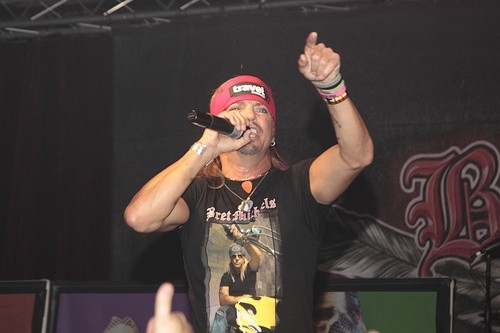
312 74 350 105
190 142 214 167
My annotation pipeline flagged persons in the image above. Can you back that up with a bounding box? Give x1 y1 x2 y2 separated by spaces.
122 31 373 333
146 282 193 333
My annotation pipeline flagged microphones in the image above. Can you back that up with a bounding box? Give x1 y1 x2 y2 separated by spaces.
475 240 500 255
188 109 250 139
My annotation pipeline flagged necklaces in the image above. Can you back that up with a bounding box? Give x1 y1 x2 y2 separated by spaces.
230 174 261 181
223 170 268 213
